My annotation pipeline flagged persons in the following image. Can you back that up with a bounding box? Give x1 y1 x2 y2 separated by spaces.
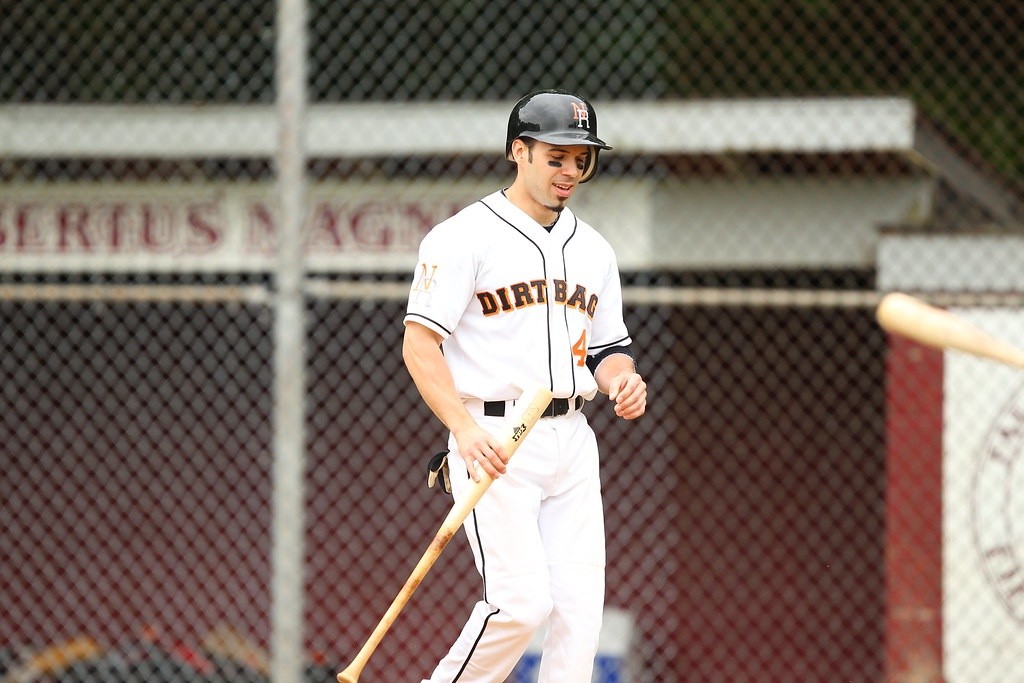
402 89 647 683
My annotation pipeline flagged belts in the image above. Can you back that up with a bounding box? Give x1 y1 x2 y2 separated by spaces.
484 395 583 418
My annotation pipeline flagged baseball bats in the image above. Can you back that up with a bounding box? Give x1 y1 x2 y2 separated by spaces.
330 384 554 683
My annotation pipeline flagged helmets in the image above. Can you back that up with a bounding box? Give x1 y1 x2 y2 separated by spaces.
505 89 614 184
